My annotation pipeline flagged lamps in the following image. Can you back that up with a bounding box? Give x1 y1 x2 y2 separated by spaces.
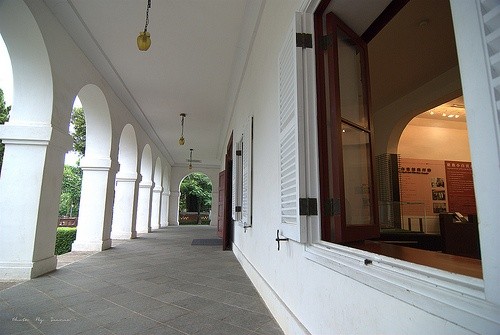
136 0 152 52
188 149 194 169
179 113 187 146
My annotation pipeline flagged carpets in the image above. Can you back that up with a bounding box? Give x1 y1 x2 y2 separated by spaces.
191 238 222 246
